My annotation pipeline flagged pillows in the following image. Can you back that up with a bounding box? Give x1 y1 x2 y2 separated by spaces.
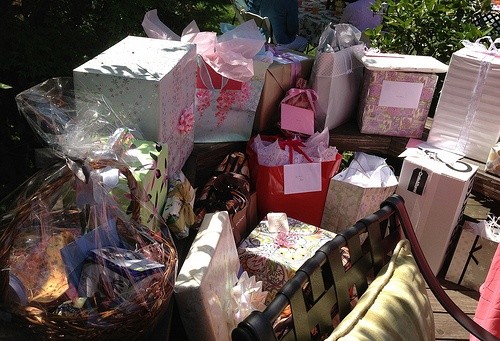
325 239 436 341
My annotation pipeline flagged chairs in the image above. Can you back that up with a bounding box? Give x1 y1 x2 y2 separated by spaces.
229 192 500 341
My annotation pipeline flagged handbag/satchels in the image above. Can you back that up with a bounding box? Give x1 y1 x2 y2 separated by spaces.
195 54 270 141
193 150 252 229
254 137 342 227
323 152 399 246
307 41 365 134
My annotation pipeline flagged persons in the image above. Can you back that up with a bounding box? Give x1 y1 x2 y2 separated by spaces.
251 0 308 53
340 0 383 32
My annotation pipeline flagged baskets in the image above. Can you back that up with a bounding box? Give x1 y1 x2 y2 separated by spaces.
0 159 177 340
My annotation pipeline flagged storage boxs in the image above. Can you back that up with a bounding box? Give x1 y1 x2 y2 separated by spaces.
360 70 438 139
427 45 500 164
90 132 168 232
484 142 500 176
384 148 479 278
72 34 197 184
174 211 244 341
444 224 500 292
79 246 166 305
258 50 315 133
237 212 337 305
